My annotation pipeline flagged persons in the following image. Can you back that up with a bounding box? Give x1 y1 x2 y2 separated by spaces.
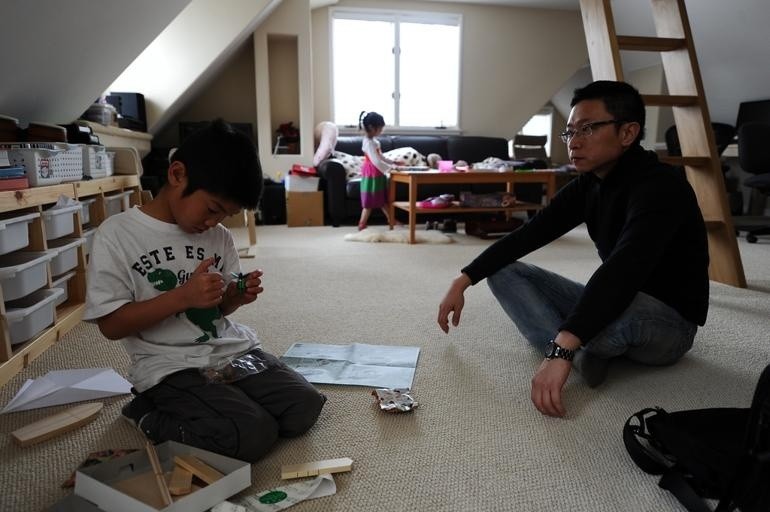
357 110 404 230
81 119 327 466
438 79 710 418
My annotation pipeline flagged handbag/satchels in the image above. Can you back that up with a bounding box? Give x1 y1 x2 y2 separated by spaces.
623 362 770 512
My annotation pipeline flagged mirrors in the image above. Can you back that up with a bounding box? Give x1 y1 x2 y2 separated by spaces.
265 29 303 156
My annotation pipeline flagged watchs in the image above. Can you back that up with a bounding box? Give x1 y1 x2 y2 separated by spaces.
543 339 575 363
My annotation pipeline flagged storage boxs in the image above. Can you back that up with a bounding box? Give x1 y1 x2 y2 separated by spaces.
106 87 146 128
283 172 321 192
286 190 325 226
2 117 134 346
91 97 114 124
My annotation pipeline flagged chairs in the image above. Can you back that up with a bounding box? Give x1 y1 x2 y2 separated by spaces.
724 94 769 244
510 132 550 166
660 116 737 161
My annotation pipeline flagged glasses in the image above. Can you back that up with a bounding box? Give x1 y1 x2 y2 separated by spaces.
560 119 618 144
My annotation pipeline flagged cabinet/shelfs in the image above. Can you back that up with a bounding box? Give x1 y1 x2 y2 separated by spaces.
1 129 144 388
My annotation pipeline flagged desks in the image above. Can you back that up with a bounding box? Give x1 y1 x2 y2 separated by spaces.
386 164 556 245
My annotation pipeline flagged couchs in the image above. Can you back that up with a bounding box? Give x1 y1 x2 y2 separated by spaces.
319 128 519 228
